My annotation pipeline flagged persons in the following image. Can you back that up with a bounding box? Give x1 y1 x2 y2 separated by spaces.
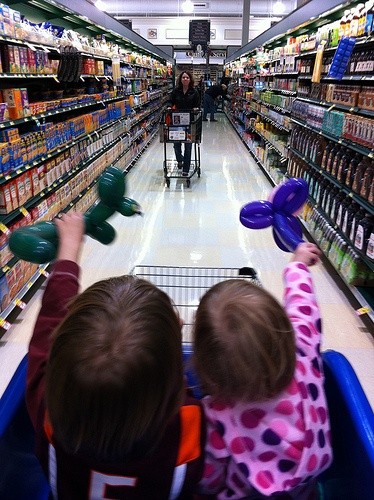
203 84 228 122
167 71 201 176
191 243 334 500
26 213 212 500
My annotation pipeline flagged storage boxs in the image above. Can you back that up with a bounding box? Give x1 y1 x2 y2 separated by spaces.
0 44 143 315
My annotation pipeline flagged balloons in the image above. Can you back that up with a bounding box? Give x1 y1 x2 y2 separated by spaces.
9 167 143 264
239 178 317 267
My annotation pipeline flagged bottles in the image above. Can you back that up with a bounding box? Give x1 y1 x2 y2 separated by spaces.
281 0 374 285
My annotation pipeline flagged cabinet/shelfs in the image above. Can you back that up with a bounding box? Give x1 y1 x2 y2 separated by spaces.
0 0 175 338
223 0 374 337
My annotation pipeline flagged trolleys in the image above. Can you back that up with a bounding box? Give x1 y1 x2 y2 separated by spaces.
0 266 374 500
159 106 206 188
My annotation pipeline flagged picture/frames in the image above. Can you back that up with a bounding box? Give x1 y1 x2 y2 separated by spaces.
147 29 157 39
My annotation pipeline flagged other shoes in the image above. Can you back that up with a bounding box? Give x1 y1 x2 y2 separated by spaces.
182 171 188 176
203 118 208 121
210 119 219 122
178 161 182 169
